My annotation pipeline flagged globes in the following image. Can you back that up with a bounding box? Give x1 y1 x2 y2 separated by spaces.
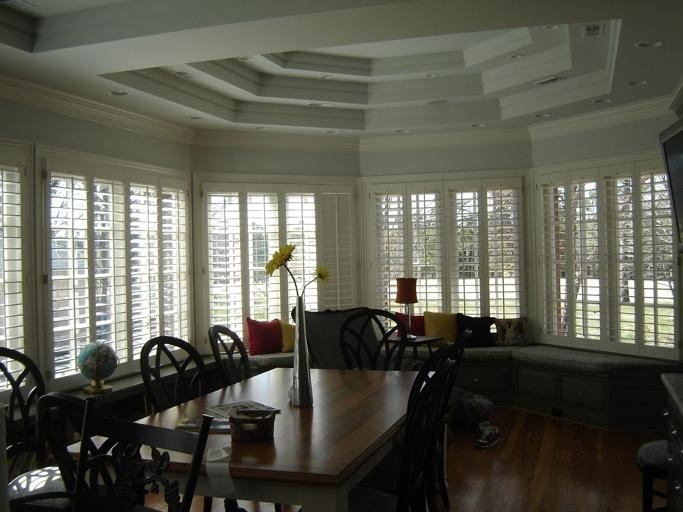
78 341 117 393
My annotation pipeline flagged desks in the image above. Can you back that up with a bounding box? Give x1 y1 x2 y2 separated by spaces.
375 333 445 370
658 374 682 512
67 369 437 512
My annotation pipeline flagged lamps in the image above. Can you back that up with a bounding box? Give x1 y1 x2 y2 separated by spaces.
395 278 419 339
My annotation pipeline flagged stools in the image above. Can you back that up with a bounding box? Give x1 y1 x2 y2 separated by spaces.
636 440 669 512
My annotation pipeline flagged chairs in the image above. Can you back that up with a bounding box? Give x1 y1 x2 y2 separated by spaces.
209 325 249 388
341 308 407 370
349 344 451 511
139 336 212 512
1 347 214 512
392 342 464 511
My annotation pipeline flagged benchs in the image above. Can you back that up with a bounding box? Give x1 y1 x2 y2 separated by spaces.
393 342 526 405
512 345 680 431
217 350 295 375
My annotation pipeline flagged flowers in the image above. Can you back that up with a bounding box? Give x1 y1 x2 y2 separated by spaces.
264 245 329 297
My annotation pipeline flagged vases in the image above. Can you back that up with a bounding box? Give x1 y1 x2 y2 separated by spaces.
292 297 312 406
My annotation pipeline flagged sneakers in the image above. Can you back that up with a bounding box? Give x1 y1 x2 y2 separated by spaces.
475 424 500 450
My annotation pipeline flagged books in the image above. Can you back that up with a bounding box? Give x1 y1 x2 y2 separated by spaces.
175 400 281 434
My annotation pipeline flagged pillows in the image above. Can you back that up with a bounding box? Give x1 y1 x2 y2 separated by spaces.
424 312 457 344
246 318 282 355
394 312 425 336
456 313 495 347
279 321 295 352
495 317 529 346
